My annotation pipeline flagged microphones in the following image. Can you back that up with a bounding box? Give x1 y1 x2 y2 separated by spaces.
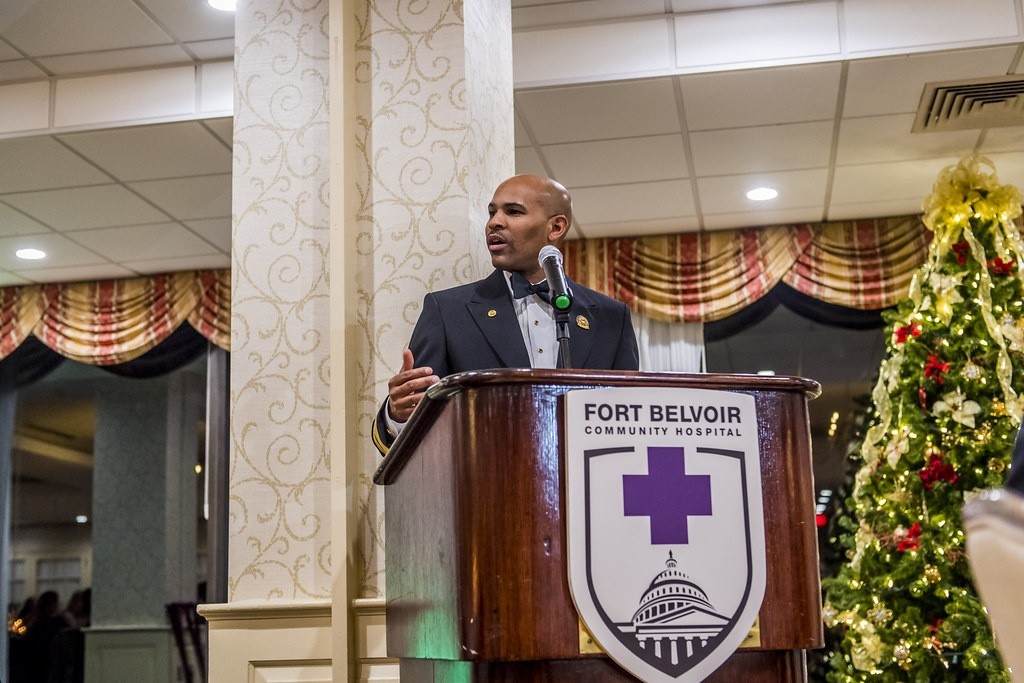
537 244 572 310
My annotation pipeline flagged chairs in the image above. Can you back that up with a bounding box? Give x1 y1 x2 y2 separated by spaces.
964 488 1024 683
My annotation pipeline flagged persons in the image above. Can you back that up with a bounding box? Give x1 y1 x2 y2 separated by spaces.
371 175 639 456
10 588 91 683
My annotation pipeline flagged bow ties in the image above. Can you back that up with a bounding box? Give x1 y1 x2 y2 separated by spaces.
512 271 550 304
